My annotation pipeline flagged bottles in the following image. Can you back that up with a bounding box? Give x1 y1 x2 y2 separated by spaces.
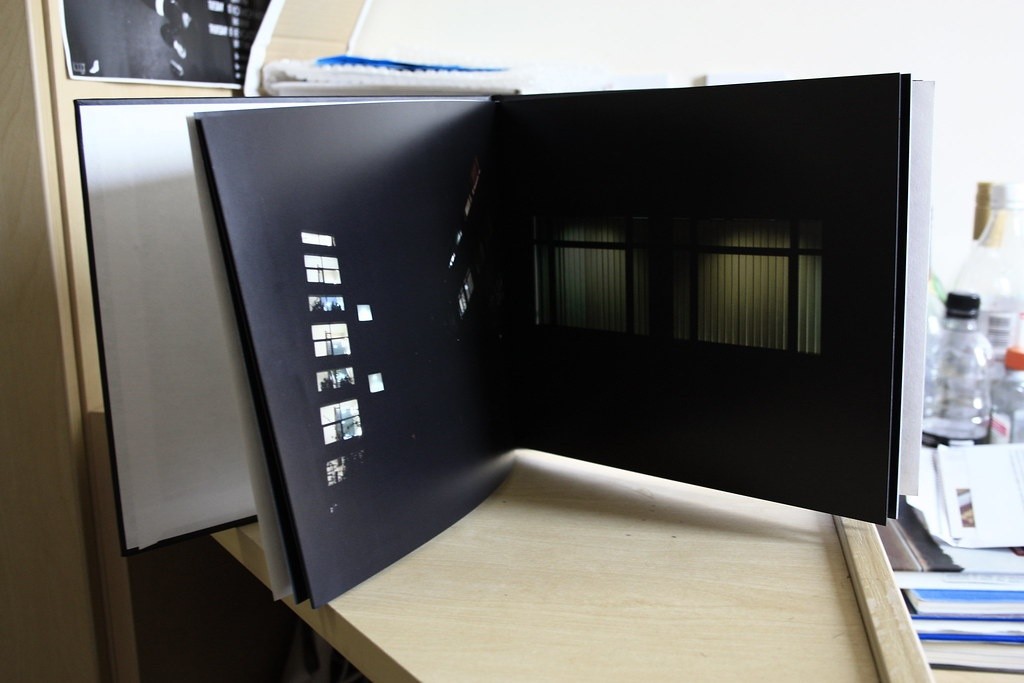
921 292 993 446
992 348 1024 444
